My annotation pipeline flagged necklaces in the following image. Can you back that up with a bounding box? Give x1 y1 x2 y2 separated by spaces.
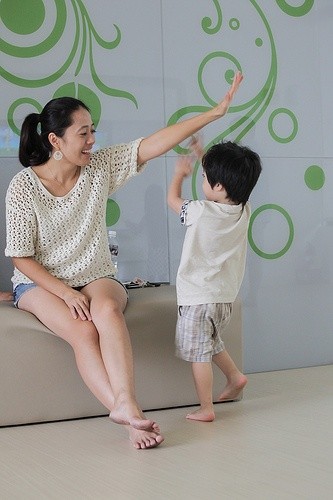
46 161 79 194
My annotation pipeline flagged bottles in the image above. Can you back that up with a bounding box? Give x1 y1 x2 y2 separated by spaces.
108 231 119 268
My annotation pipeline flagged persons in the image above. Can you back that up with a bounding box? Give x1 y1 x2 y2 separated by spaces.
167 135 262 421
6 69 244 449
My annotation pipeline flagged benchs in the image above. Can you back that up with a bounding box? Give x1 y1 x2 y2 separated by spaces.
0 283 242 428
